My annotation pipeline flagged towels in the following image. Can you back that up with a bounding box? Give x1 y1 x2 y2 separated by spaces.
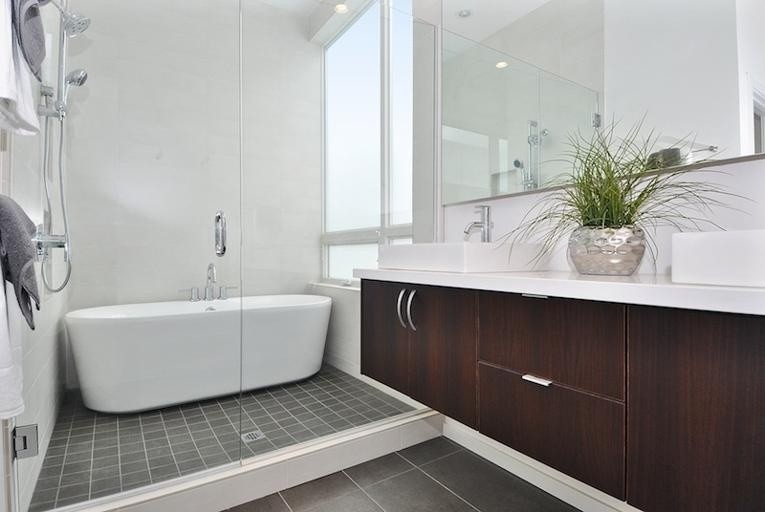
1 0 55 139
0 194 42 421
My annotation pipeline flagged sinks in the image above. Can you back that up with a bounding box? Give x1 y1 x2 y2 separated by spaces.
671 230 765 288
378 241 548 274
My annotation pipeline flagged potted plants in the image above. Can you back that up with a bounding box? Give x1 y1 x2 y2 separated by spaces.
494 110 759 277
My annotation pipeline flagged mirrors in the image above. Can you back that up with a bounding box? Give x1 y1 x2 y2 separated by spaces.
438 3 765 208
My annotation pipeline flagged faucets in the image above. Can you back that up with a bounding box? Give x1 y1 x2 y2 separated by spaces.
207 263 216 287
464 206 489 242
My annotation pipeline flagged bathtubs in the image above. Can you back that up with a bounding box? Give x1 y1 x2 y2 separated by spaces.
64 294 332 414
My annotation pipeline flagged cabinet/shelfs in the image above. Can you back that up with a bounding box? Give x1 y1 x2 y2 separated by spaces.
478 290 628 501
628 304 762 512
360 280 478 434
